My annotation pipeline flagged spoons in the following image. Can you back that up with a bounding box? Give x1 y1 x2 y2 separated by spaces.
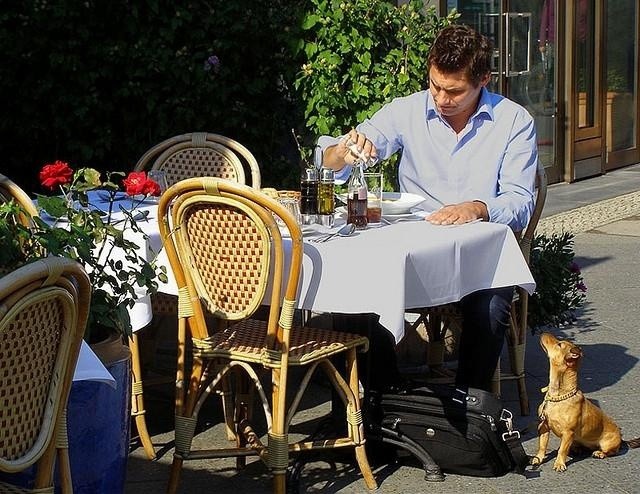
110 209 149 223
319 222 356 243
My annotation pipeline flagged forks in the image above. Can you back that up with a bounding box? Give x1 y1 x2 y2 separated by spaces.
381 215 425 225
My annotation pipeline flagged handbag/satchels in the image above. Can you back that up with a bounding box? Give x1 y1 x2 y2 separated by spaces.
362 382 530 479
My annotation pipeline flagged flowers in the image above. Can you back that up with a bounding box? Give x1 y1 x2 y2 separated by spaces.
1 140 182 345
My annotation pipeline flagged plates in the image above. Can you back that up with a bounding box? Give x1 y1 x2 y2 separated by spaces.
130 194 165 205
337 190 427 214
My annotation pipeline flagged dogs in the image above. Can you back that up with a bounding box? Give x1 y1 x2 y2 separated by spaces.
528 331 640 472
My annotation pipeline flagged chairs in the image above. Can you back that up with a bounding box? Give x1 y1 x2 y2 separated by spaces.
1 176 381 494
399 152 548 415
129 130 263 190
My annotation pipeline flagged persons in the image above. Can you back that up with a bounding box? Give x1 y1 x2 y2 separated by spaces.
312 23 540 425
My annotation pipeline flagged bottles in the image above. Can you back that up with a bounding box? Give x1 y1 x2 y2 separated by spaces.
300 167 320 226
347 159 368 230
319 168 337 229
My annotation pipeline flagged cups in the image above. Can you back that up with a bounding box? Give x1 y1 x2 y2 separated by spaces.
363 173 384 228
145 169 168 196
